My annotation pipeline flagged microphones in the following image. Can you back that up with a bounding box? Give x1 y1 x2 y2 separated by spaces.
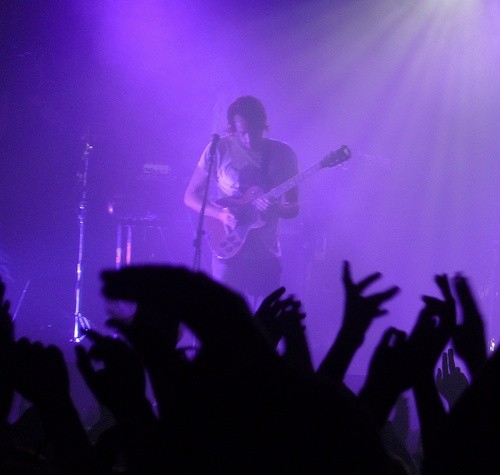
207 133 221 162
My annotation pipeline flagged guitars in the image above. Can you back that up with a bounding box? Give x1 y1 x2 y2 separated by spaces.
204 144 352 261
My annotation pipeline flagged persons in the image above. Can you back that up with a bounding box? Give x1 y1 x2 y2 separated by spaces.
0 263 500 474
182 95 300 318
